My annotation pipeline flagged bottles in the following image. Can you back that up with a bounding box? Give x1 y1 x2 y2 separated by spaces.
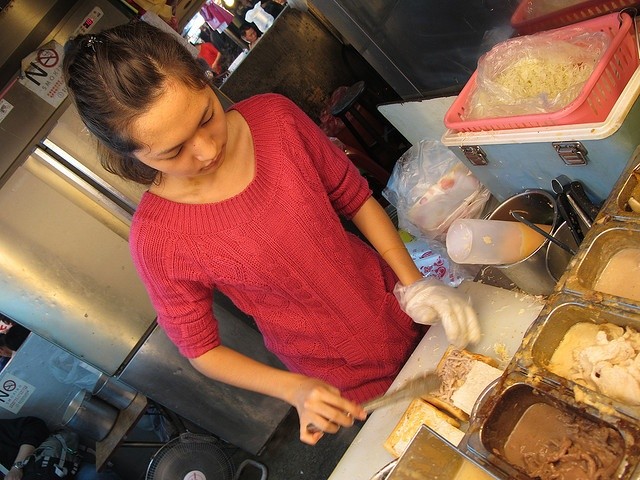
446 217 552 264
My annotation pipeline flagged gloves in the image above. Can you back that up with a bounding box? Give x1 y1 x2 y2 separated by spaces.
394 278 483 349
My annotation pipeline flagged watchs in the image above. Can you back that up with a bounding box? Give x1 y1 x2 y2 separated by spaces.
12 461 23 470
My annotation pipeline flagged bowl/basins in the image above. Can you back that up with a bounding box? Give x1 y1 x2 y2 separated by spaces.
507 292 640 424
454 369 639 476
554 222 640 316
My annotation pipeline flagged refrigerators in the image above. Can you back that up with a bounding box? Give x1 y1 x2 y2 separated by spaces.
1 0 296 458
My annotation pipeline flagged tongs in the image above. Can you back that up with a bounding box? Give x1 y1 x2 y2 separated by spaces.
305 370 443 434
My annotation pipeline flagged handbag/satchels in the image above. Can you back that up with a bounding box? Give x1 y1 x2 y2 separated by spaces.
198 1 235 34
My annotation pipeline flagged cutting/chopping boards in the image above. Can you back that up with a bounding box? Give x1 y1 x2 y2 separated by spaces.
328 280 547 476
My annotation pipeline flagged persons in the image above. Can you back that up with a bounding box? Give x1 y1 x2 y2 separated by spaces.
63 22 479 446
189 41 221 75
239 24 262 49
0 418 48 480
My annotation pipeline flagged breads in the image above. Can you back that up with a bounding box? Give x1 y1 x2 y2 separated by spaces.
422 343 508 424
382 396 465 461
